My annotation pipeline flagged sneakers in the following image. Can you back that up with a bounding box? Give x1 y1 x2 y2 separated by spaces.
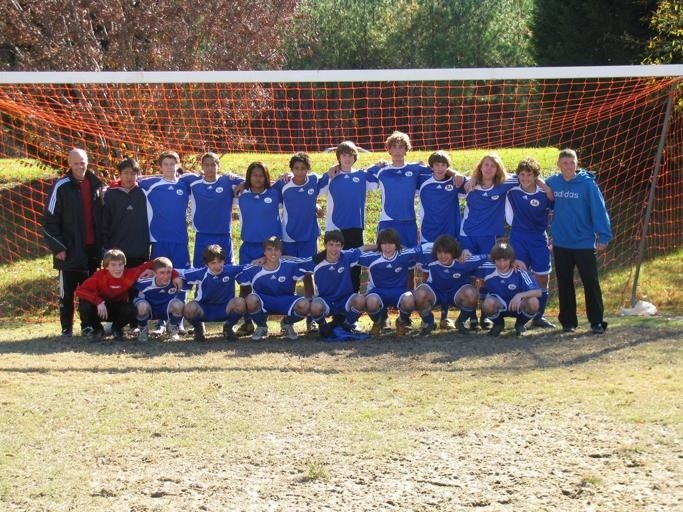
562 326 575 333
237 324 253 335
592 323 603 334
368 319 453 339
223 326 234 336
304 316 363 338
62 329 71 337
81 327 205 343
281 322 297 339
251 327 267 340
456 316 556 335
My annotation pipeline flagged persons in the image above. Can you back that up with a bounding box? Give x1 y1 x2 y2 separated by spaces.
187 152 246 335
98 150 237 336
544 146 615 333
74 248 184 344
227 230 555 341
93 157 152 338
130 257 190 342
226 130 556 332
41 147 108 339
137 243 296 340
